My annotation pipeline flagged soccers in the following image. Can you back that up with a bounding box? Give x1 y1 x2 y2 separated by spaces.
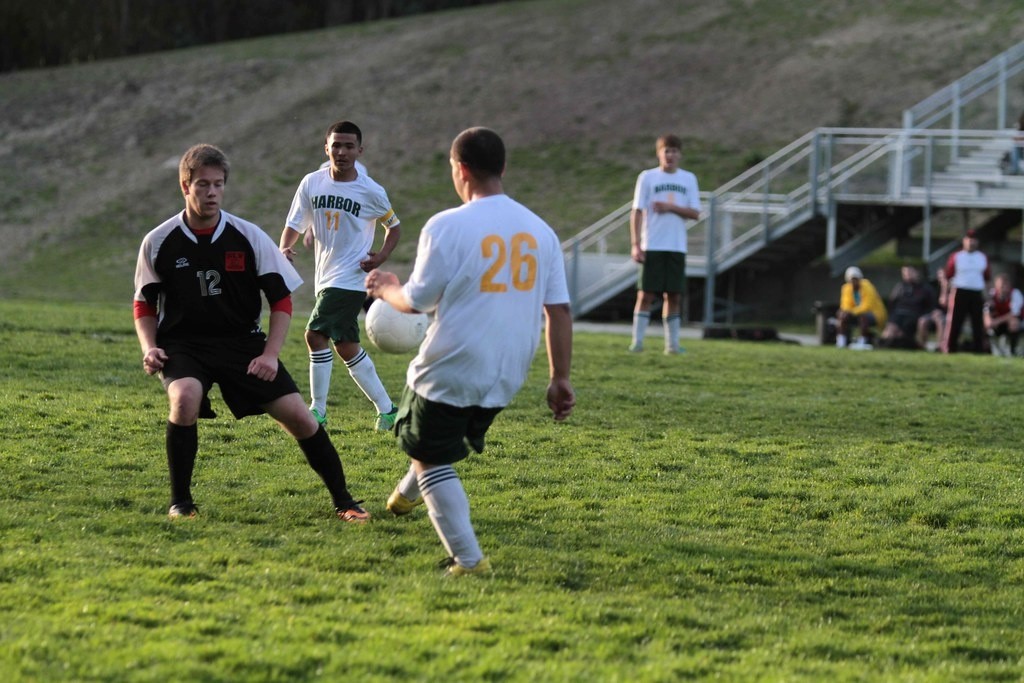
365 298 428 353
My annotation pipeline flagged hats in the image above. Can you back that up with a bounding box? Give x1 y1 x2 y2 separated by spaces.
962 228 980 241
844 266 864 283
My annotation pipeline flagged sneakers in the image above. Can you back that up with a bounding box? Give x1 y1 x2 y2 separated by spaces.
432 552 495 584
374 404 401 436
309 405 329 431
337 502 376 528
385 475 443 518
167 498 200 527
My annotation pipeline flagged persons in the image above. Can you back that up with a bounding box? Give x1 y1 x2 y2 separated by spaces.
365 126 578 578
629 134 705 354
278 121 402 434
835 227 1024 356
133 143 374 523
